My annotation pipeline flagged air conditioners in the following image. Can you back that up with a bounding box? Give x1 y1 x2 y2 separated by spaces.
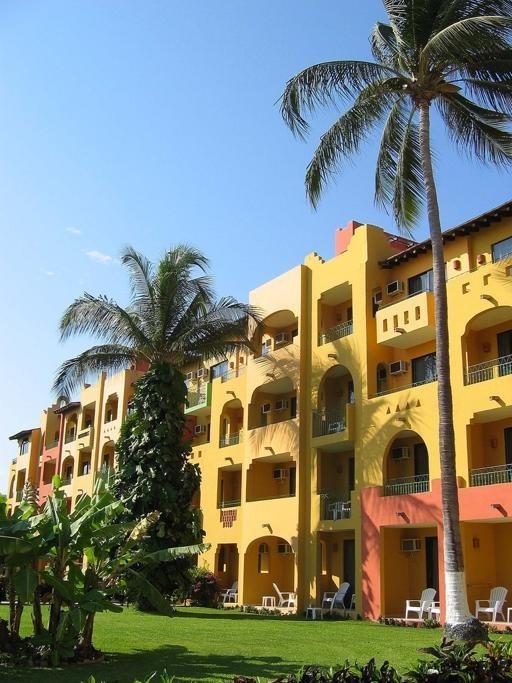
263 398 288 414
265 331 289 349
194 424 206 434
186 367 208 380
390 446 411 460
272 468 287 480
399 537 422 553
375 358 407 381
373 278 402 304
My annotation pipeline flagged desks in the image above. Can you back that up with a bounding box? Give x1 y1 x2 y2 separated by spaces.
262 595 276 607
305 607 323 621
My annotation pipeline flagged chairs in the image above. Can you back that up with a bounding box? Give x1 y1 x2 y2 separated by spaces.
272 582 294 608
322 581 356 609
217 579 238 603
474 586 508 622
406 586 440 621
326 499 351 521
